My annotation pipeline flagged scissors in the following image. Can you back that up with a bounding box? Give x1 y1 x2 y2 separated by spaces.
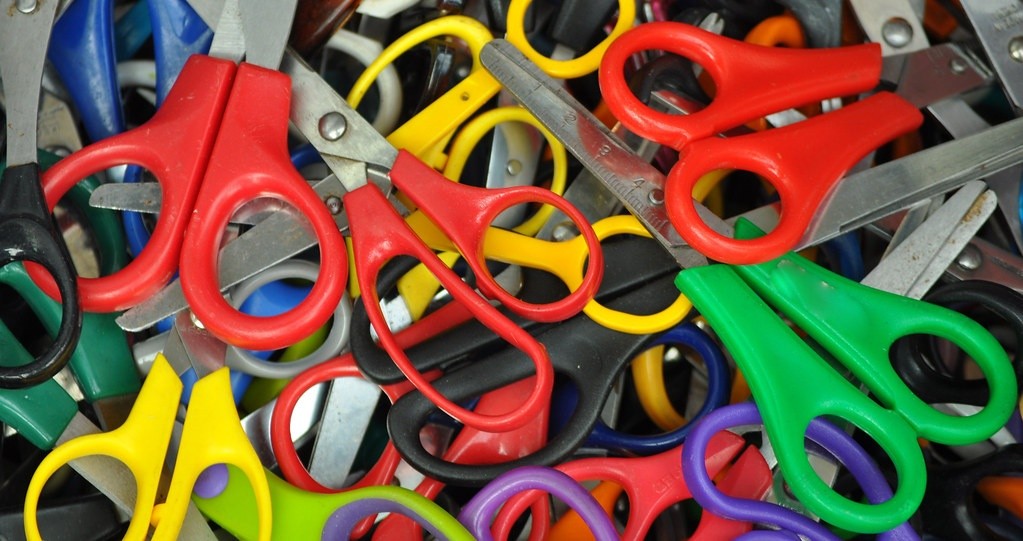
0 0 1022 541
